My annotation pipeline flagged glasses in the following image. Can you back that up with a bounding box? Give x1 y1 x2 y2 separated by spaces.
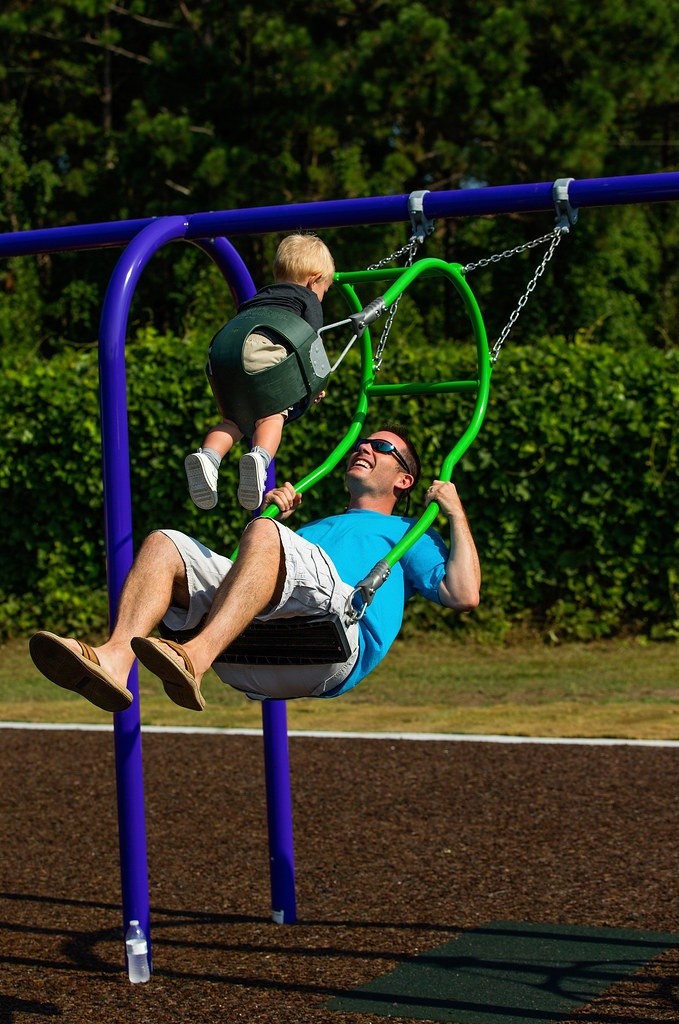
356 438 411 475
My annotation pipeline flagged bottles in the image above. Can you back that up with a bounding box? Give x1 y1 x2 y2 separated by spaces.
125 920 150 984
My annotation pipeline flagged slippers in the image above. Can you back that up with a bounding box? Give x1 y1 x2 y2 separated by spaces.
131 637 207 711
29 631 134 712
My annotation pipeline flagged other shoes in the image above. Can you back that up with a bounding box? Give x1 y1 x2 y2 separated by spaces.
185 447 219 509
238 446 268 510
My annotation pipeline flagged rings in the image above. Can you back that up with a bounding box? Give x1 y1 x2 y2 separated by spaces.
429 486 433 493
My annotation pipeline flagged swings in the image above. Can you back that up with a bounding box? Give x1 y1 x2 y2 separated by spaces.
158 178 580 665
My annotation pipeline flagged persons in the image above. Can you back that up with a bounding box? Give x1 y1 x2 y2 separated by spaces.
183 231 335 511
28 424 482 712
